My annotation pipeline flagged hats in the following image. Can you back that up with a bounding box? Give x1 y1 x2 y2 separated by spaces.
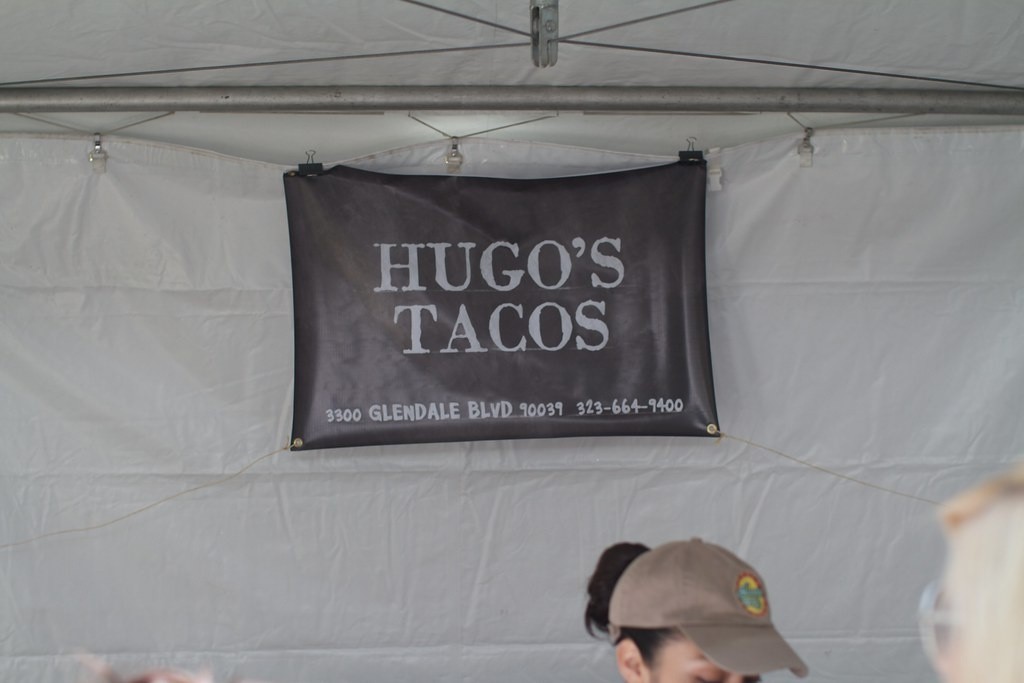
608 537 809 679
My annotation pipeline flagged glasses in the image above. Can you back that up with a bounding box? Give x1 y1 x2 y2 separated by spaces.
919 586 963 662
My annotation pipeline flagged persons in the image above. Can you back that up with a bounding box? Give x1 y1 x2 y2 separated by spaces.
585 537 808 683
